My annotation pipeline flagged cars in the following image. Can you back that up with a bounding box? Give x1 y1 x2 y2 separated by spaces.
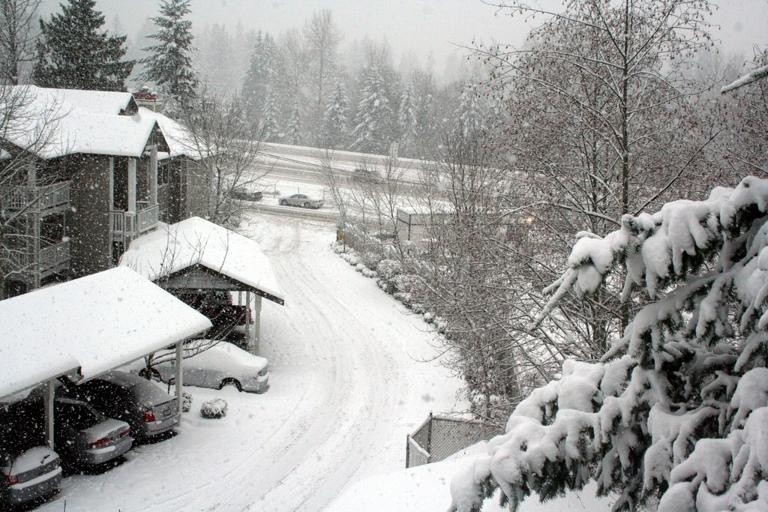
0 369 181 512
278 193 325 209
222 186 263 203
353 168 383 183
128 339 271 395
